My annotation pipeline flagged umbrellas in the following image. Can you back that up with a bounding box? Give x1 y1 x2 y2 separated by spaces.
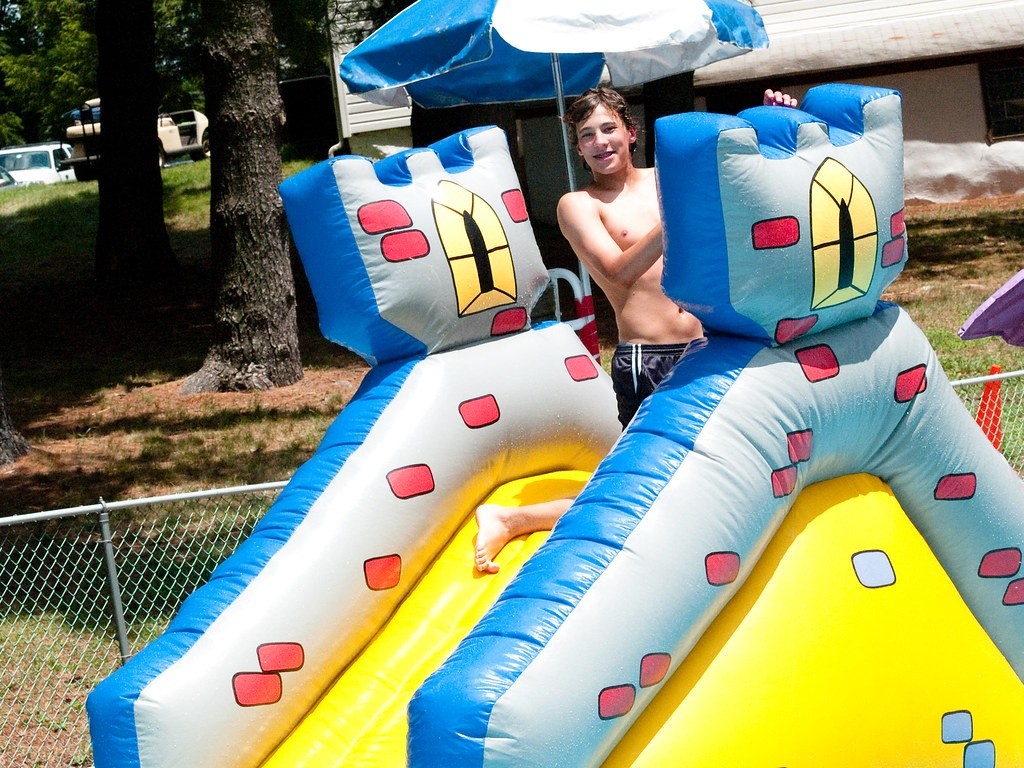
338 0 769 366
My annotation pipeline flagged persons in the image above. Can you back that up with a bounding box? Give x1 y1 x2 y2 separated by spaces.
472 86 797 573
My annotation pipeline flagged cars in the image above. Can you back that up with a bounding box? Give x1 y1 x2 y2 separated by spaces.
0 142 76 191
